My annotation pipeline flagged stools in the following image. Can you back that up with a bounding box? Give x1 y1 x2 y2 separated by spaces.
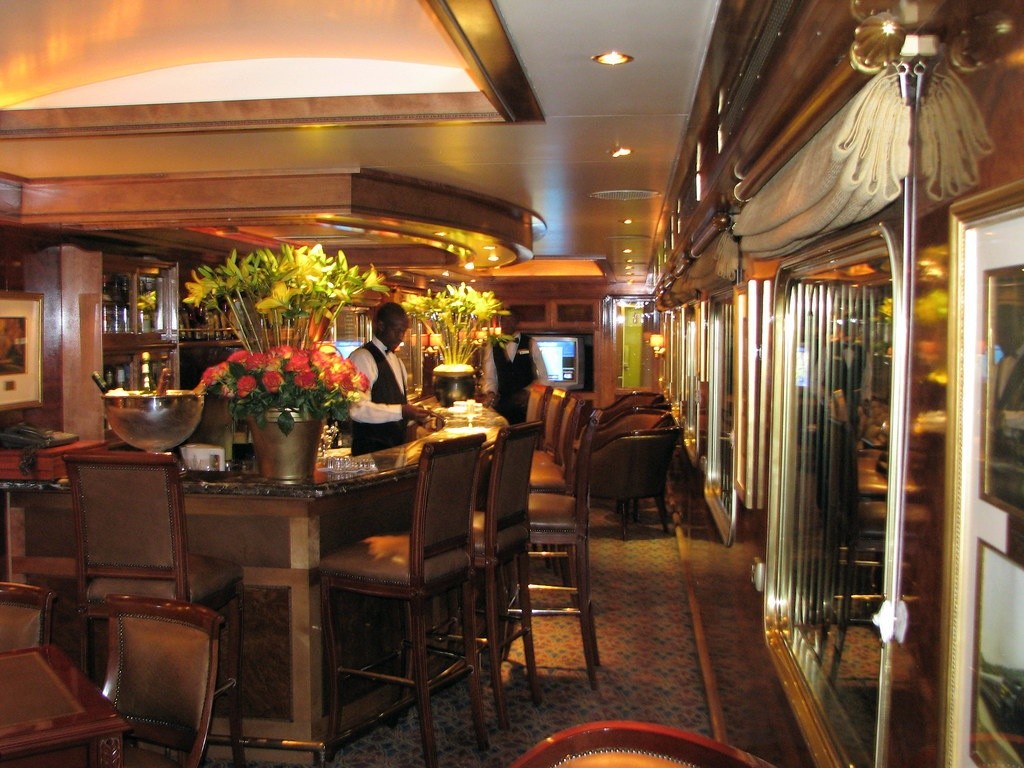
0 383 770 768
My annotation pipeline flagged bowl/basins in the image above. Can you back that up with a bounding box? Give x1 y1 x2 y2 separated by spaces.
102 389 204 452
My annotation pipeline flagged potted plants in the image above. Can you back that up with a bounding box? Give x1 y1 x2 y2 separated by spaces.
400 281 516 410
183 243 393 479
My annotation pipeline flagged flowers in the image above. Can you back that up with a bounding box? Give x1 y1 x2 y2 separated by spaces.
194 342 373 437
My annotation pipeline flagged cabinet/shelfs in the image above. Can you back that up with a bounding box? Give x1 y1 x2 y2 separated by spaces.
103 255 233 439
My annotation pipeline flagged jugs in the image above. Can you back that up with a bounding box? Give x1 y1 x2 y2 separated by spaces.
179 443 226 474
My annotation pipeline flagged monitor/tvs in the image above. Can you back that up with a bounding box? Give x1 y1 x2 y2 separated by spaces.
532 336 579 385
334 341 362 361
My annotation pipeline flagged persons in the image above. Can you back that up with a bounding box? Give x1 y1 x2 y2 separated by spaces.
839 344 861 410
482 309 548 427
995 304 1024 464
345 302 429 457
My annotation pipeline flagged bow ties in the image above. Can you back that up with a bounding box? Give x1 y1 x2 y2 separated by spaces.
504 338 519 344
385 346 394 355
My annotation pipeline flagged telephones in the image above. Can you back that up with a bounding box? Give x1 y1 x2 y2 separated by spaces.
0 422 80 447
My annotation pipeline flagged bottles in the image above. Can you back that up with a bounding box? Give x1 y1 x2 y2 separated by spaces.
153 368 172 396
102 274 236 341
93 372 113 396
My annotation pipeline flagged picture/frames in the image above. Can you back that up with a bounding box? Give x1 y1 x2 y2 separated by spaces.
0 290 44 411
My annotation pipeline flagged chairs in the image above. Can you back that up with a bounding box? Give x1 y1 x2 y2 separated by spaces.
589 425 683 541
592 406 679 449
601 392 666 425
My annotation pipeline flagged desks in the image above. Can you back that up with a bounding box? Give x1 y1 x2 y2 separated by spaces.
0 645 134 768
